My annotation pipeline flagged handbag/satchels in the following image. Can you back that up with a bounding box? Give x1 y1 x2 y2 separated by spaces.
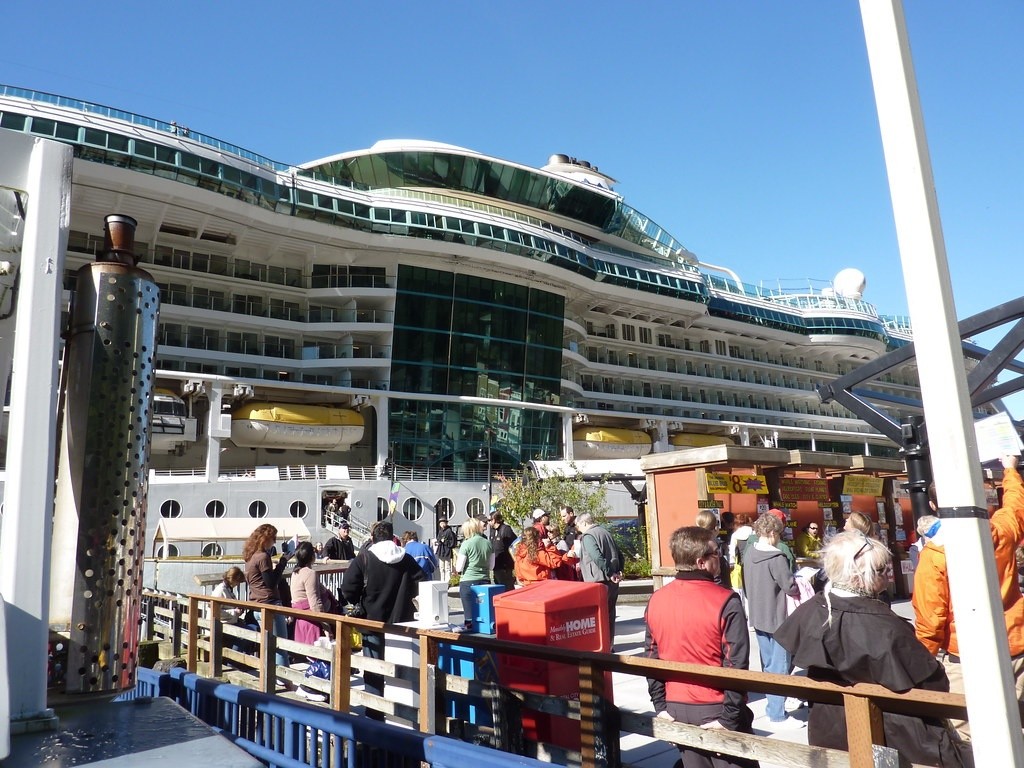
272 578 290 600
245 609 259 626
730 555 742 588
351 627 362 652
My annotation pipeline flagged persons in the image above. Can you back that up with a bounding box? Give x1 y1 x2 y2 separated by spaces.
643 526 760 768
290 542 327 701
910 515 942 591
741 509 795 628
322 495 351 530
794 522 825 559
206 567 260 668
839 510 874 542
774 529 974 768
720 512 736 545
310 507 585 615
912 451 1024 743
342 521 429 719
245 524 294 692
574 512 625 653
696 510 733 591
451 517 496 633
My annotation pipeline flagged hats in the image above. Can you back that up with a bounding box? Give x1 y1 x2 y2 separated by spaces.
439 516 449 522
770 509 786 524
475 514 490 522
339 522 352 530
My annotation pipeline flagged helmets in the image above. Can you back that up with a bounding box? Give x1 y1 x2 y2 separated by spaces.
532 508 551 521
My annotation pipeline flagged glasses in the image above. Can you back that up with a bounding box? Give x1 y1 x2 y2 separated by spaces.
854 535 871 559
483 521 487 523
704 549 722 557
878 568 894 577
809 527 819 530
548 531 554 533
488 519 493 521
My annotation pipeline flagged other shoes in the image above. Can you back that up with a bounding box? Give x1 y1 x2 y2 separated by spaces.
295 687 328 701
461 627 472 633
770 716 804 729
276 678 287 686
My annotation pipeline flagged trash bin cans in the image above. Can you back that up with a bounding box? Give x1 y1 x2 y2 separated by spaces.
436 583 539 760
385 581 457 732
491 578 615 754
